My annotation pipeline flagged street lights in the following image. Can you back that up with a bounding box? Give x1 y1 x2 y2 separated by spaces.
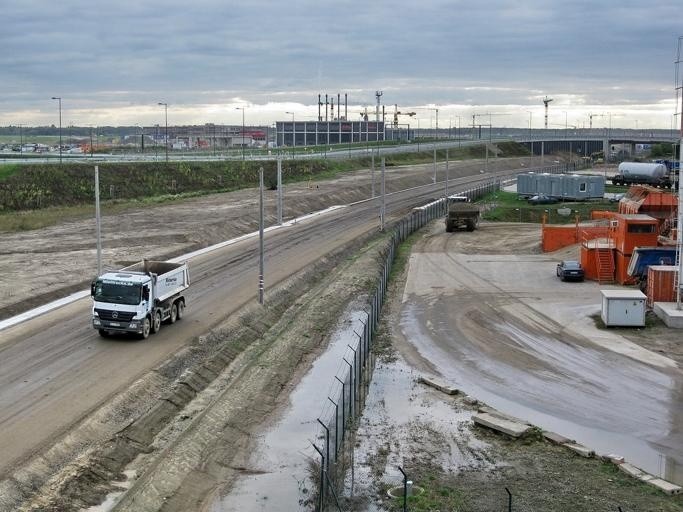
562 111 567 128
157 102 169 162
606 111 611 128
235 107 244 159
525 110 532 139
50 96 62 164
285 111 294 159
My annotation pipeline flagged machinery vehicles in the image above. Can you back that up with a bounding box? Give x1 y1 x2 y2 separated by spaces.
609 160 671 189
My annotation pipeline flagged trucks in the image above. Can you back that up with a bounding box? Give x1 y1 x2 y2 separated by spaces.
88 256 193 342
441 193 480 234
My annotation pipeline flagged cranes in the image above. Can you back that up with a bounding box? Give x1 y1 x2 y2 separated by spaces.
357 106 387 121
383 102 418 128
541 94 553 127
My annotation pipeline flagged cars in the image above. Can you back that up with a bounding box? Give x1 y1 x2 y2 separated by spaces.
0 139 83 155
246 138 275 148
526 193 557 205
554 258 585 284
